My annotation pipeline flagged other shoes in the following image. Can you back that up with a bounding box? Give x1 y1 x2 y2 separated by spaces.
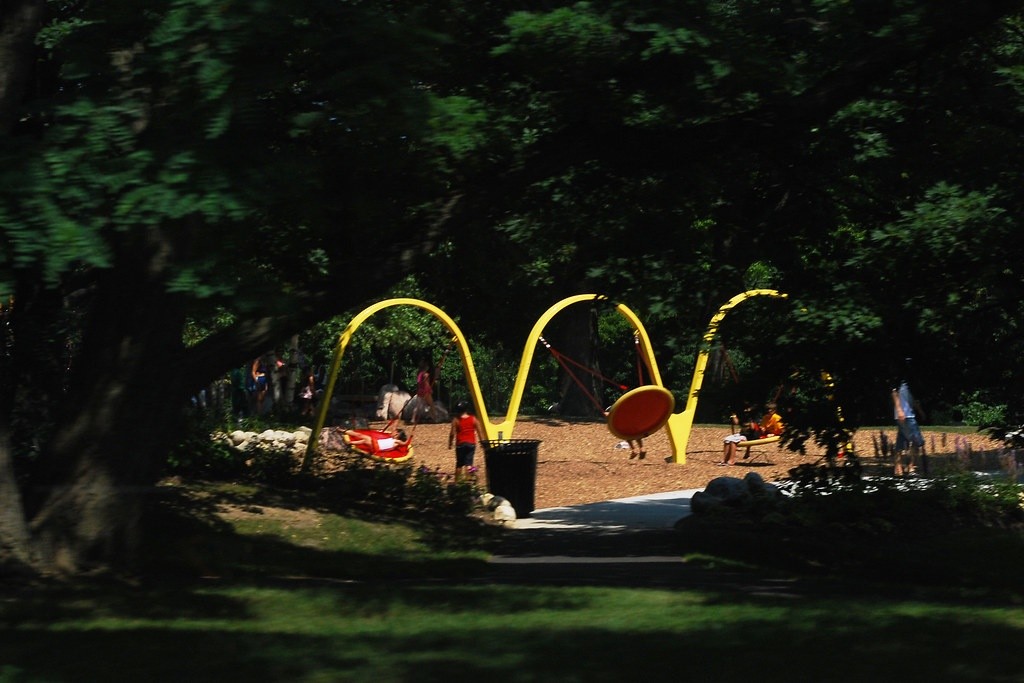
629 451 638 459
717 462 727 466
639 448 646 459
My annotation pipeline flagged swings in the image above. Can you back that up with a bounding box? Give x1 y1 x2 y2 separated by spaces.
715 332 802 446
337 335 459 462
539 330 674 442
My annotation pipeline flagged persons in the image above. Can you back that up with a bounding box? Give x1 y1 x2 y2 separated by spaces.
890 379 924 478
628 438 646 460
409 363 439 423
449 400 483 482
712 401 786 466
252 359 328 416
335 426 414 450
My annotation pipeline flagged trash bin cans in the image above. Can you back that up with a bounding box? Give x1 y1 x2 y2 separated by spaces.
480 439 542 518
